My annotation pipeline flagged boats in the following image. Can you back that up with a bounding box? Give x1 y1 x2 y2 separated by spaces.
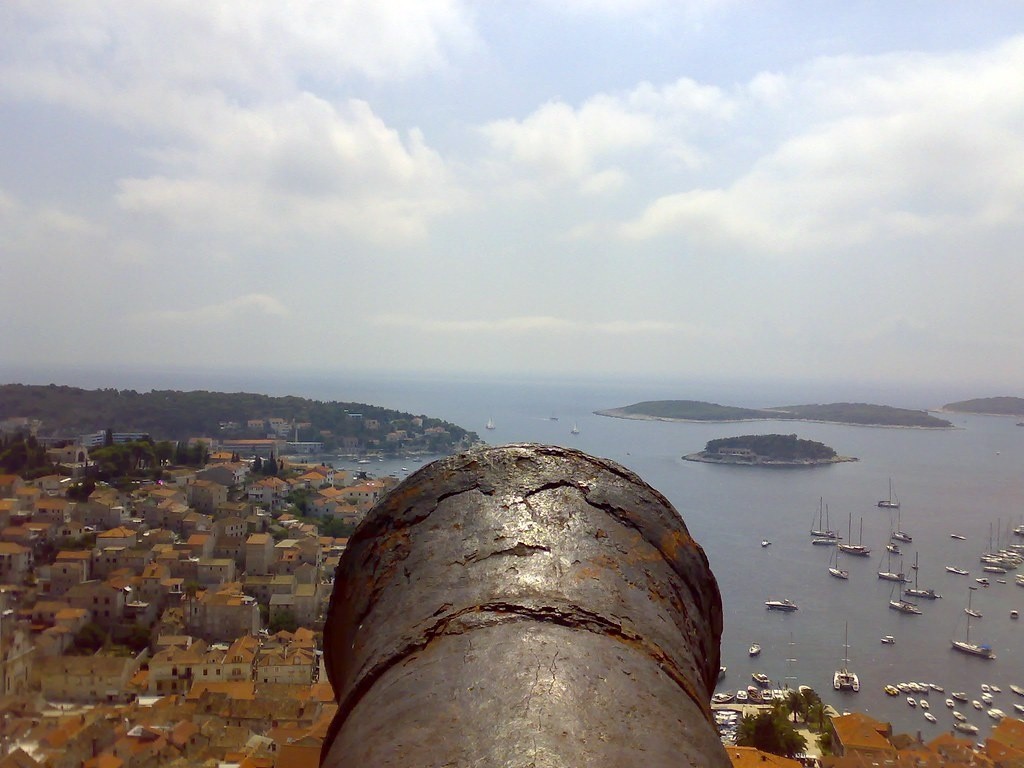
353 456 423 478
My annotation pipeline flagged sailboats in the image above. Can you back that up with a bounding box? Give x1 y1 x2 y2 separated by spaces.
712 472 1024 747
486 417 495 429
572 420 579 434
